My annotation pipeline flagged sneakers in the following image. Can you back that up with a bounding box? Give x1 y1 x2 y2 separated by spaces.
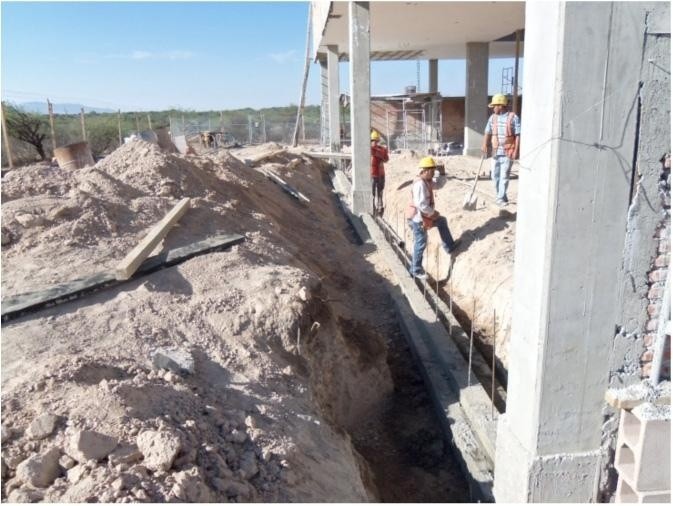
413 270 430 280
446 240 461 253
496 196 509 205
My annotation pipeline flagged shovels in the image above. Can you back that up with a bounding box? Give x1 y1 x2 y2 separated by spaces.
463 153 485 209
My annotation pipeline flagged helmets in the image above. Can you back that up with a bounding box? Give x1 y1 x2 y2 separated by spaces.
371 131 380 140
488 94 508 108
419 157 436 167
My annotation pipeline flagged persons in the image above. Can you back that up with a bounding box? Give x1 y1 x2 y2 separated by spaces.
405 154 462 281
480 93 520 207
369 129 388 215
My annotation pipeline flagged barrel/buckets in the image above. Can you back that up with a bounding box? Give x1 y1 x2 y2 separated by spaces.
53 141 95 171
